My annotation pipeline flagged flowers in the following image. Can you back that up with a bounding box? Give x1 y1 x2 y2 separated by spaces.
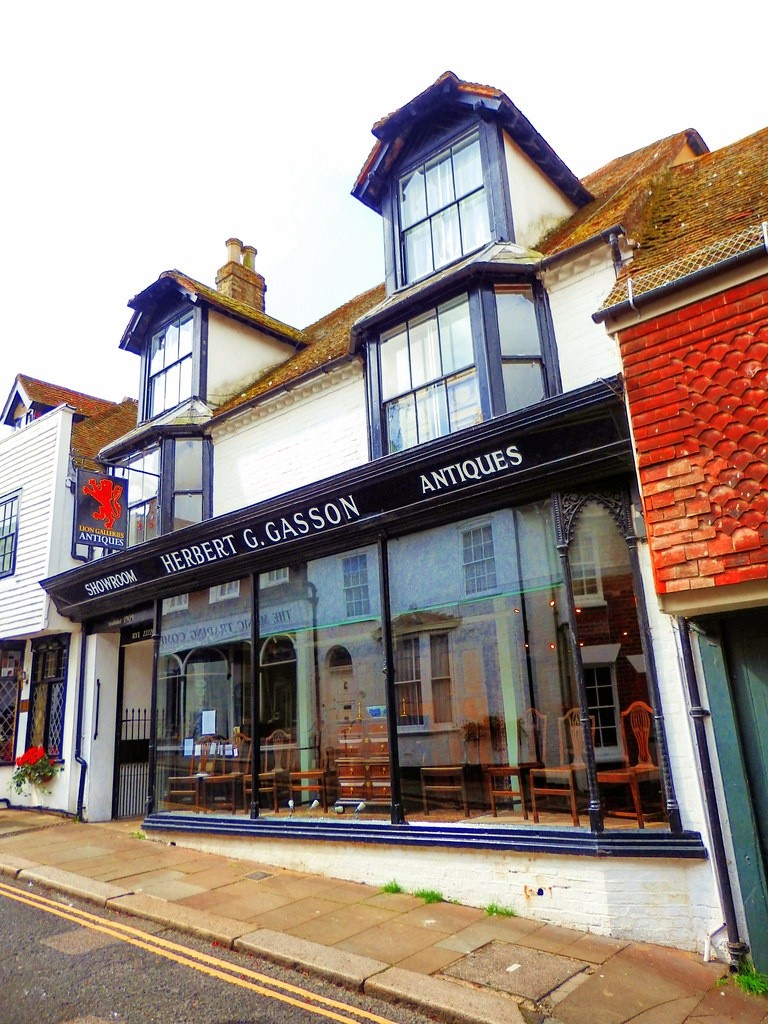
12 747 59 797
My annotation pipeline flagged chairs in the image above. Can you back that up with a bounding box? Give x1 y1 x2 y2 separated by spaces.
168 700 665 830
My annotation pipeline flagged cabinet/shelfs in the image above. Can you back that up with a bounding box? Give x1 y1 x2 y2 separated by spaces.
336 718 392 809
